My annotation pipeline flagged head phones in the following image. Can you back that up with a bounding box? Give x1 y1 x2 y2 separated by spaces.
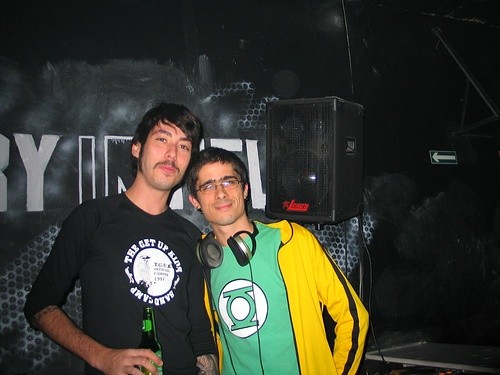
195 221 259 270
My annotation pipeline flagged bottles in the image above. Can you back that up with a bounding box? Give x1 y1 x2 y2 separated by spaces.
135 308 162 375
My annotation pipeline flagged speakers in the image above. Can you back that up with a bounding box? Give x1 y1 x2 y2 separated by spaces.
264 95 365 225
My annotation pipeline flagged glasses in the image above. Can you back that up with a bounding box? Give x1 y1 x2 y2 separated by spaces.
195 179 243 193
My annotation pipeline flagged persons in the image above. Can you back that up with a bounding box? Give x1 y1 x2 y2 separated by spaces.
186 146 369 375
23 102 219 375
